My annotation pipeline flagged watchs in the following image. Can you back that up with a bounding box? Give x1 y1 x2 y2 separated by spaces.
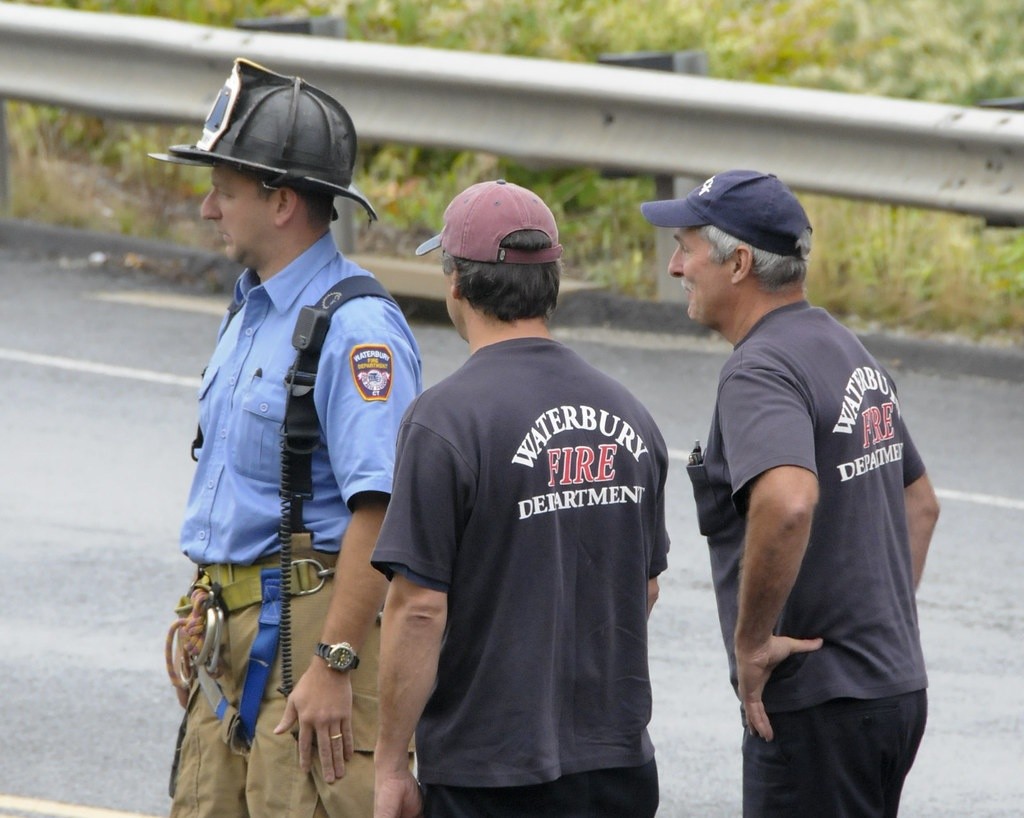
316 641 360 671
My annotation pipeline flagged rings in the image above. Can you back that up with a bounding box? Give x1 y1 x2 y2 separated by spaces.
331 732 343 739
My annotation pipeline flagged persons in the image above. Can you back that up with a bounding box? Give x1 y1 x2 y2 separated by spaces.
367 179 670 818
144 58 423 817
640 169 940 818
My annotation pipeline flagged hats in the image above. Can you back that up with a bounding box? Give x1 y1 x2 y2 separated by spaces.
415 179 563 264
641 169 812 258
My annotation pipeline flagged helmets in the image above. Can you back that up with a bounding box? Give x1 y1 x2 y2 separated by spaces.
146 56 379 220
